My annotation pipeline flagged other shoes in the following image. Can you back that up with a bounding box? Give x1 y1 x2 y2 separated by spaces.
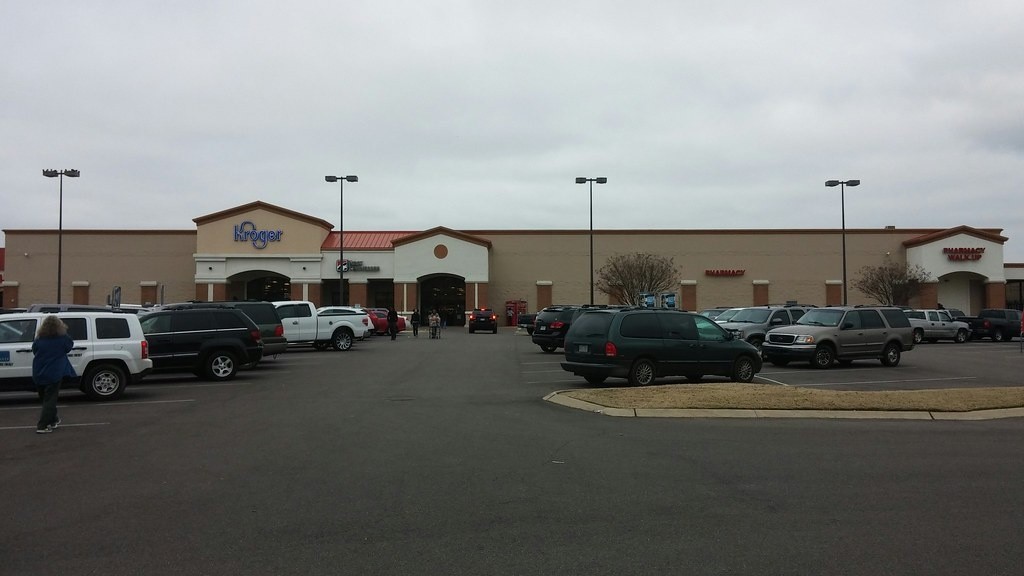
36 427 53 434
51 418 61 429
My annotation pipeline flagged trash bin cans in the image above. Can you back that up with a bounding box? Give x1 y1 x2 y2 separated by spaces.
464 311 473 327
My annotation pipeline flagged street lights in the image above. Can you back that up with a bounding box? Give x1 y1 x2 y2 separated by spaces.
43 168 81 304
576 176 608 306
824 179 859 306
324 175 358 306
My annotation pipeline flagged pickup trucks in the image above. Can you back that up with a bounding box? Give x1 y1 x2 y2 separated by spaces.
272 300 368 351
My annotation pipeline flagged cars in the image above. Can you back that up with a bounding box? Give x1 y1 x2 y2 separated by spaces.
0 300 413 342
527 303 1023 361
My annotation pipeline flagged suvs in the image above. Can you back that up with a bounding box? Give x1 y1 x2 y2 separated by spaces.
468 307 499 333
190 301 289 369
559 305 764 387
1 312 153 402
138 305 264 381
762 306 915 367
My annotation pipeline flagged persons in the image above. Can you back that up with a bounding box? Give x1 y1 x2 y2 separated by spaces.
440 307 448 329
428 309 439 339
387 305 398 340
507 308 514 326
31 316 76 433
411 309 420 336
